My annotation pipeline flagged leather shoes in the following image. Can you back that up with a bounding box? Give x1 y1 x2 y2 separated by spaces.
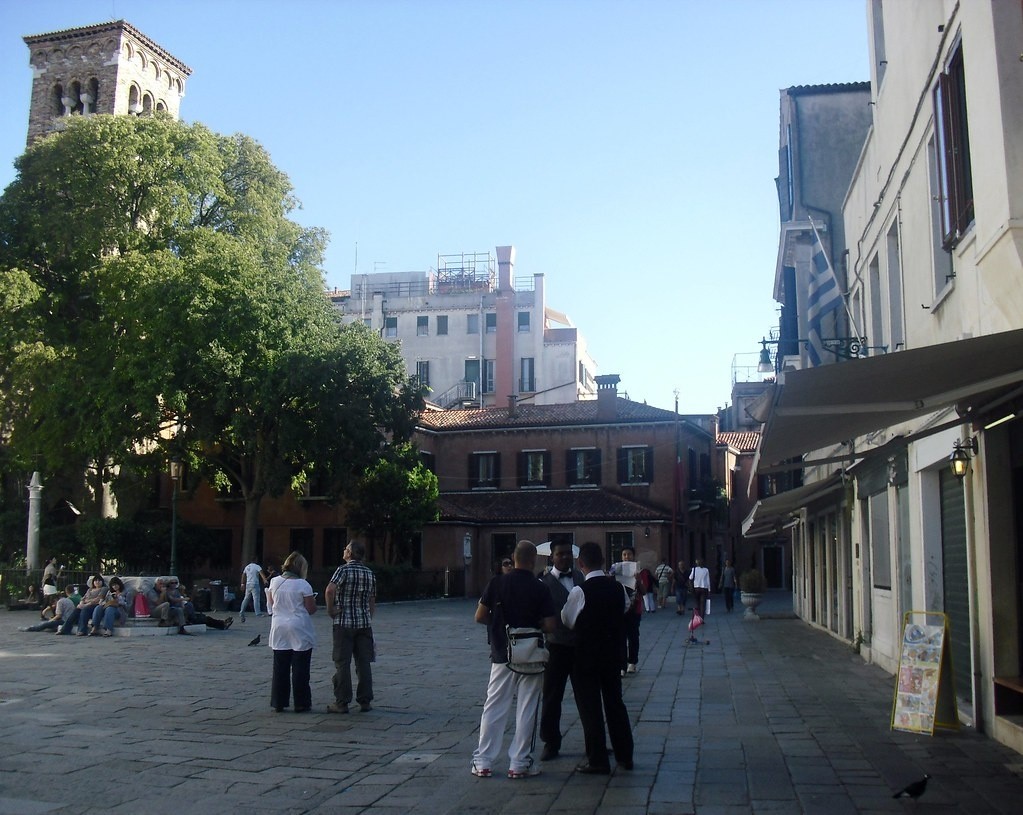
576 761 610 774
613 749 634 770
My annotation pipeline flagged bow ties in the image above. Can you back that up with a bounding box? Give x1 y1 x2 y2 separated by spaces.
559 572 573 579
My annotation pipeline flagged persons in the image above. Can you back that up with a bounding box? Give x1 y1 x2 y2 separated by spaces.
669 559 689 615
166 577 192 634
40 555 63 620
642 569 659 613
55 574 109 636
178 585 192 625
184 592 234 630
88 577 132 637
17 594 75 631
717 558 738 614
688 553 711 624
540 538 612 761
25 584 41 609
536 556 553 578
325 538 376 713
238 555 269 622
655 556 673 607
66 584 82 606
147 577 170 627
268 551 317 713
471 540 555 779
490 553 513 576
610 544 649 677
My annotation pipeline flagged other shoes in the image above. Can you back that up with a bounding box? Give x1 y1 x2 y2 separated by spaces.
360 702 372 712
676 610 686 615
225 615 234 630
179 629 190 634
275 705 283 712
540 745 559 761
294 705 312 712
646 610 650 613
326 701 349 714
55 630 113 637
158 621 171 626
627 663 636 672
621 670 626 677
652 610 656 613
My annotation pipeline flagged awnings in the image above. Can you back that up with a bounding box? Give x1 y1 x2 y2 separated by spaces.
742 468 850 538
747 329 1023 499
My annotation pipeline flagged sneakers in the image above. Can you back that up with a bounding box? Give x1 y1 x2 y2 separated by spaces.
508 758 543 779
471 761 492 777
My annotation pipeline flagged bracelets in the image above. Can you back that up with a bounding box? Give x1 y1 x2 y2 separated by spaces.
241 584 245 586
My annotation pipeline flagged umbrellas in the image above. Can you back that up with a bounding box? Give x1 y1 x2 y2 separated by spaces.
536 542 579 558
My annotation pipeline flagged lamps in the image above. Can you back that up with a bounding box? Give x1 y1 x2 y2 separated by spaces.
950 435 978 484
757 335 866 373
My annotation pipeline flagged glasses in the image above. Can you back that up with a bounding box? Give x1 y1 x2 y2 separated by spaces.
501 560 514 567
170 581 179 584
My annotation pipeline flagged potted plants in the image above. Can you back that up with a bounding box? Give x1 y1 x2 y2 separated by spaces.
737 568 766 618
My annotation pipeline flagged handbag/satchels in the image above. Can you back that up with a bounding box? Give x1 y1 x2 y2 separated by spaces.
704 596 711 615
689 579 695 592
721 575 725 586
506 625 550 675
654 579 659 588
132 592 151 618
